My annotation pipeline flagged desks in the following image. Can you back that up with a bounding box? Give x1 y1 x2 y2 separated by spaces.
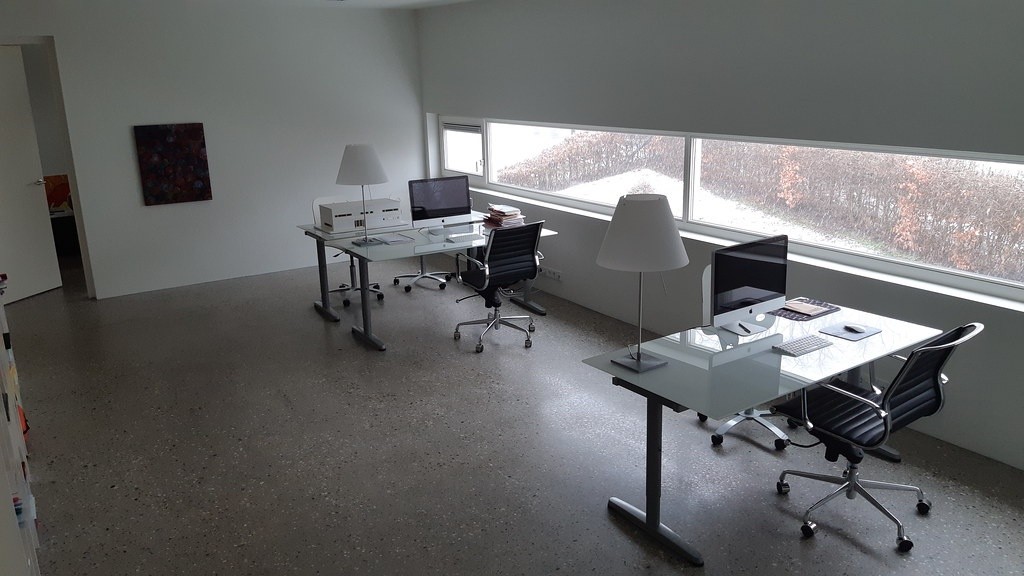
295 210 491 322
583 295 944 566
324 223 559 352
50 209 78 269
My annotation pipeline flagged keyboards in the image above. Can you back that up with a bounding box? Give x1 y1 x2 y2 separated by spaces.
446 234 484 243
772 335 833 357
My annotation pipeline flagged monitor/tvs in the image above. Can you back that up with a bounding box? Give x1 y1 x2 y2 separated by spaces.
408 175 472 237
710 235 788 337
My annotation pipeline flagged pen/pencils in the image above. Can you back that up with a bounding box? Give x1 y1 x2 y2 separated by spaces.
739 322 751 333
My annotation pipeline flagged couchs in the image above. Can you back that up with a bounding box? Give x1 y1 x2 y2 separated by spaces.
43 175 72 209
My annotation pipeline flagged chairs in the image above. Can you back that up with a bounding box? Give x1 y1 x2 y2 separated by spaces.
695 263 798 452
777 321 985 553
392 255 452 293
454 219 545 353
312 195 386 307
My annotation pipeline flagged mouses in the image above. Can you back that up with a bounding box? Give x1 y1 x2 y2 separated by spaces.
845 325 866 333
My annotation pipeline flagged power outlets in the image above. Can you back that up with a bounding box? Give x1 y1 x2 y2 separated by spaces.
836 372 890 396
538 265 563 283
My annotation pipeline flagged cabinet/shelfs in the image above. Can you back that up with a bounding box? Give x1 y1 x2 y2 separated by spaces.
0 296 43 576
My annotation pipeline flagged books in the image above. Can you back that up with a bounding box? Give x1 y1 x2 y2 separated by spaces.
785 300 829 316
375 234 413 245
483 203 526 228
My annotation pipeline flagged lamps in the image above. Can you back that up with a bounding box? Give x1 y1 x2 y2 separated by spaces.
336 143 389 248
593 193 690 373
